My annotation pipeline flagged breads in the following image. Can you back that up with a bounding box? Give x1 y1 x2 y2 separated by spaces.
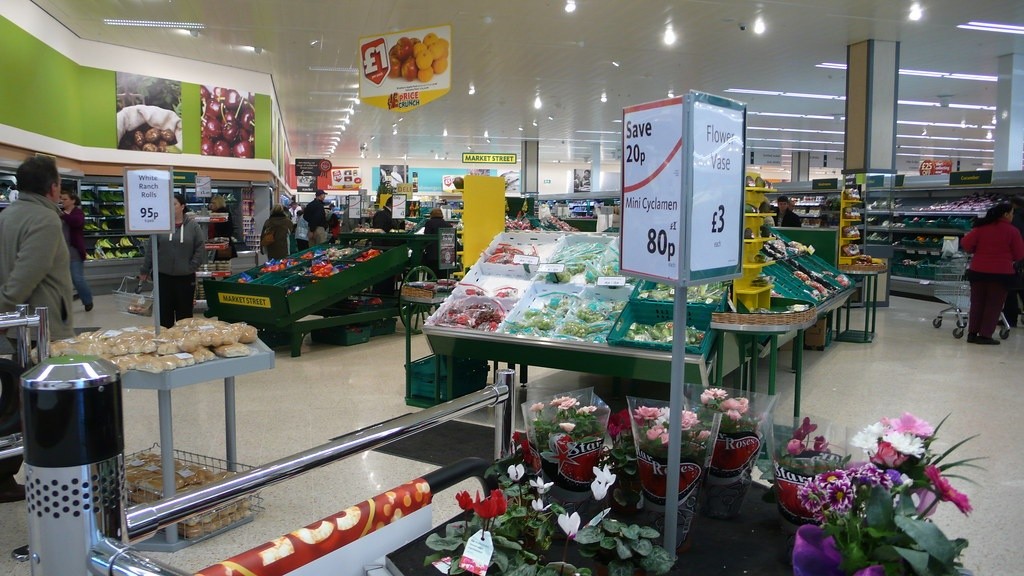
126 452 251 540
30 317 259 373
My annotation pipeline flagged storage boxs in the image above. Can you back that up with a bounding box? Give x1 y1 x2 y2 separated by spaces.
223 245 391 295
312 318 398 346
389 218 425 234
892 216 976 280
404 353 488 401
424 229 856 362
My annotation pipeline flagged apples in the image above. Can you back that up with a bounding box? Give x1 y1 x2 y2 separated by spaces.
387 36 421 83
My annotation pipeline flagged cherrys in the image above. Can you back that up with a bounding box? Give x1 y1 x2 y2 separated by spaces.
200 85 255 157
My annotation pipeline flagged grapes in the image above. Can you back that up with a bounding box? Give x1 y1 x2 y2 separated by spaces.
506 251 634 339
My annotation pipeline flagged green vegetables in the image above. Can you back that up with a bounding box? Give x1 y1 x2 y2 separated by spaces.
624 282 726 345
116 71 182 119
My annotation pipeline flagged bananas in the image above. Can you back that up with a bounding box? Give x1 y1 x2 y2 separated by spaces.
81 188 141 261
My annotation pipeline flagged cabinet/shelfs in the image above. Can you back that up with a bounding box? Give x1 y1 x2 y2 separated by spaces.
838 168 897 309
449 175 505 278
716 172 804 428
121 338 276 553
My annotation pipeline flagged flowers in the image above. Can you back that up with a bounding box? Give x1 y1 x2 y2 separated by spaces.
422 389 991 576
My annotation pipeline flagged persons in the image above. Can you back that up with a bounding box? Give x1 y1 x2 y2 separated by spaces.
304 190 328 246
771 196 801 227
59 191 93 312
0 154 73 503
580 170 590 187
140 193 206 329
327 203 340 239
959 197 1024 345
425 208 453 234
206 195 238 280
373 197 398 233
259 202 309 262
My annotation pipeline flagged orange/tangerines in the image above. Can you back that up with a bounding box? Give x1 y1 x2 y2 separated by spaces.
412 33 450 82
355 249 379 261
238 278 247 283
259 251 338 283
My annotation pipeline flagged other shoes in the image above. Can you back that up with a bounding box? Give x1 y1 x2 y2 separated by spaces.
0 481 25 503
966 333 976 343
84 301 94 311
976 335 1002 345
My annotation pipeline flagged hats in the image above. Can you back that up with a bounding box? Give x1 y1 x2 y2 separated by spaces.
314 190 330 195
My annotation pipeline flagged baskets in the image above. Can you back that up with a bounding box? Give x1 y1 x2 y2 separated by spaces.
113 275 154 318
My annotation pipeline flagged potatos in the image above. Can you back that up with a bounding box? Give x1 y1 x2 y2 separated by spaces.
119 127 176 151
